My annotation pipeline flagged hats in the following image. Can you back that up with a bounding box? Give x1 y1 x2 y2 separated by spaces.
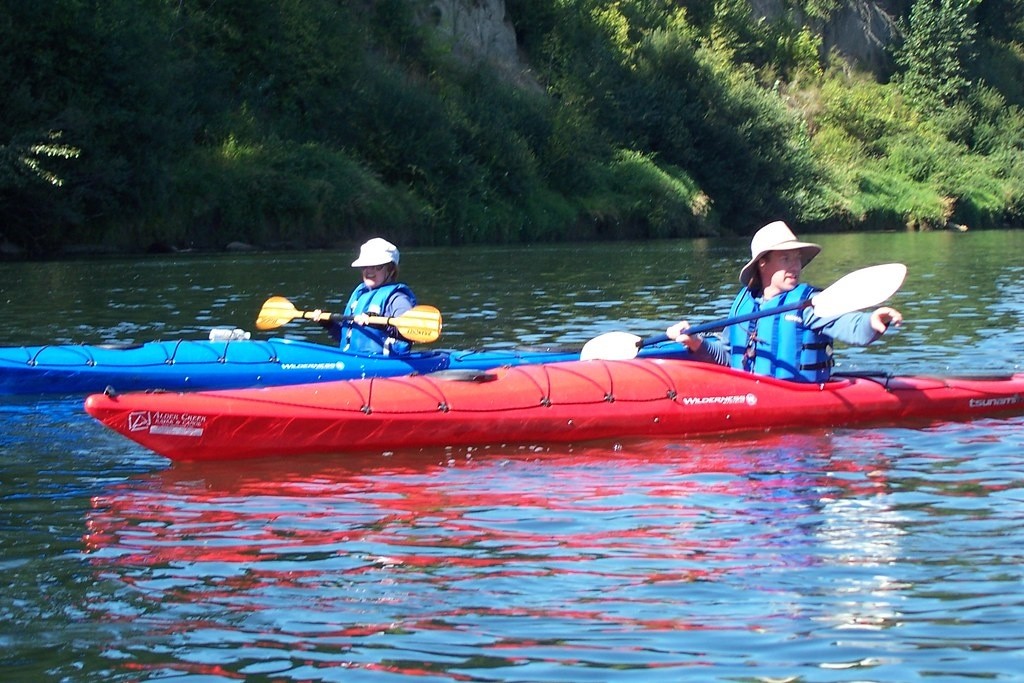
351 238 400 267
738 221 822 290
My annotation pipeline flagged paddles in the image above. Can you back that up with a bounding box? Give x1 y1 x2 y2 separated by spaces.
254 293 445 345
577 261 909 363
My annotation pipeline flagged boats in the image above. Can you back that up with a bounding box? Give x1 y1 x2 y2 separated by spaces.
84 354 1024 466
0 334 722 396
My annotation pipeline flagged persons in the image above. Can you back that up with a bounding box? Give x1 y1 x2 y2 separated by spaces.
665 220 904 385
312 236 417 357
